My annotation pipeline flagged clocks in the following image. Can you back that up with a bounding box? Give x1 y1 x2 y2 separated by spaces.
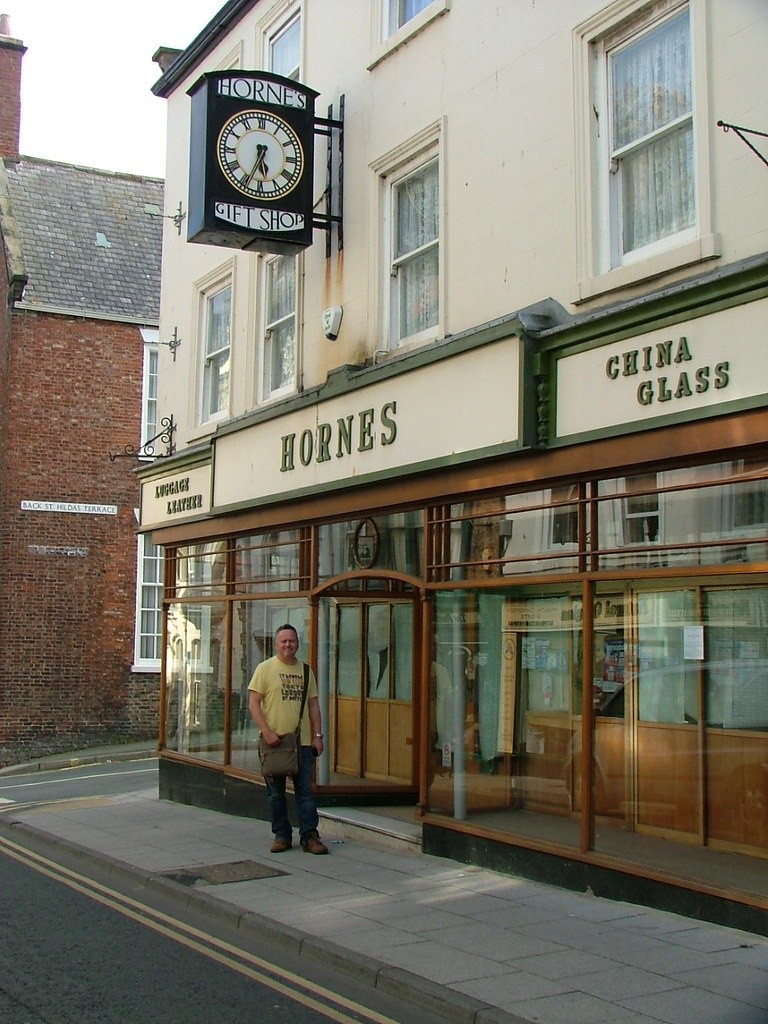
186 69 347 260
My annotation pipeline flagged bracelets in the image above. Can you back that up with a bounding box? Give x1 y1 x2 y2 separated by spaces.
313 734 323 739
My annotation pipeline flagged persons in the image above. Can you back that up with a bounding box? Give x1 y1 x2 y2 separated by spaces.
247 624 329 855
431 633 452 750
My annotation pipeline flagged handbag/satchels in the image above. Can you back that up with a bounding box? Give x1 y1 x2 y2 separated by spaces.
258 730 298 776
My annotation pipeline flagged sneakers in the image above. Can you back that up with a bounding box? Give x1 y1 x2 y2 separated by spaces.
303 835 328 855
270 832 292 852
